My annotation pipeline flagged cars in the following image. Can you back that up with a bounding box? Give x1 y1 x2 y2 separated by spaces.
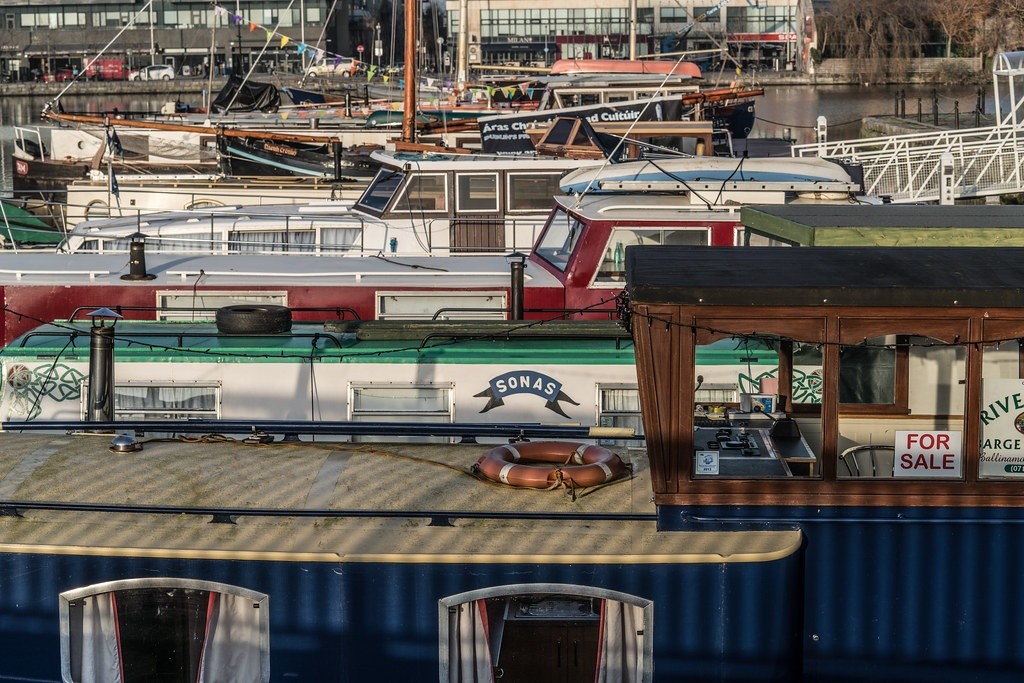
128 64 176 81
34 70 76 85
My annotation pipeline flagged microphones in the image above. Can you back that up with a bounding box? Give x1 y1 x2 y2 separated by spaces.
695 375 703 391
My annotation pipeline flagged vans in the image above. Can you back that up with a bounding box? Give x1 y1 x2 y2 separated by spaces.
305 58 354 77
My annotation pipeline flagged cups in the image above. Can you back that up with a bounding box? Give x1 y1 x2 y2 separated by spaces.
740 393 751 412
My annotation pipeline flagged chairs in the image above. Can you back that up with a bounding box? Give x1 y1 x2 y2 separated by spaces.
839 443 896 477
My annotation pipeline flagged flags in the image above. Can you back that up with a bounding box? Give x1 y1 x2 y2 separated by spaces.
106 129 124 163
111 166 119 198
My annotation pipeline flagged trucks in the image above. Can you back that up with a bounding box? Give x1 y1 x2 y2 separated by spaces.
84 57 138 82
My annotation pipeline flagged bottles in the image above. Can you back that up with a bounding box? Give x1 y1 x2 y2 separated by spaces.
614 243 620 263
620 243 624 262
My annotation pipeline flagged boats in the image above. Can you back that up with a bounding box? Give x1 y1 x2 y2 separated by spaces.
0 202 748 349
0 203 1024 445
0 246 1023 682
0 1 798 259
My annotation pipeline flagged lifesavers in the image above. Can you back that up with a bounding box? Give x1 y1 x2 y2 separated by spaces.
477 439 625 489
